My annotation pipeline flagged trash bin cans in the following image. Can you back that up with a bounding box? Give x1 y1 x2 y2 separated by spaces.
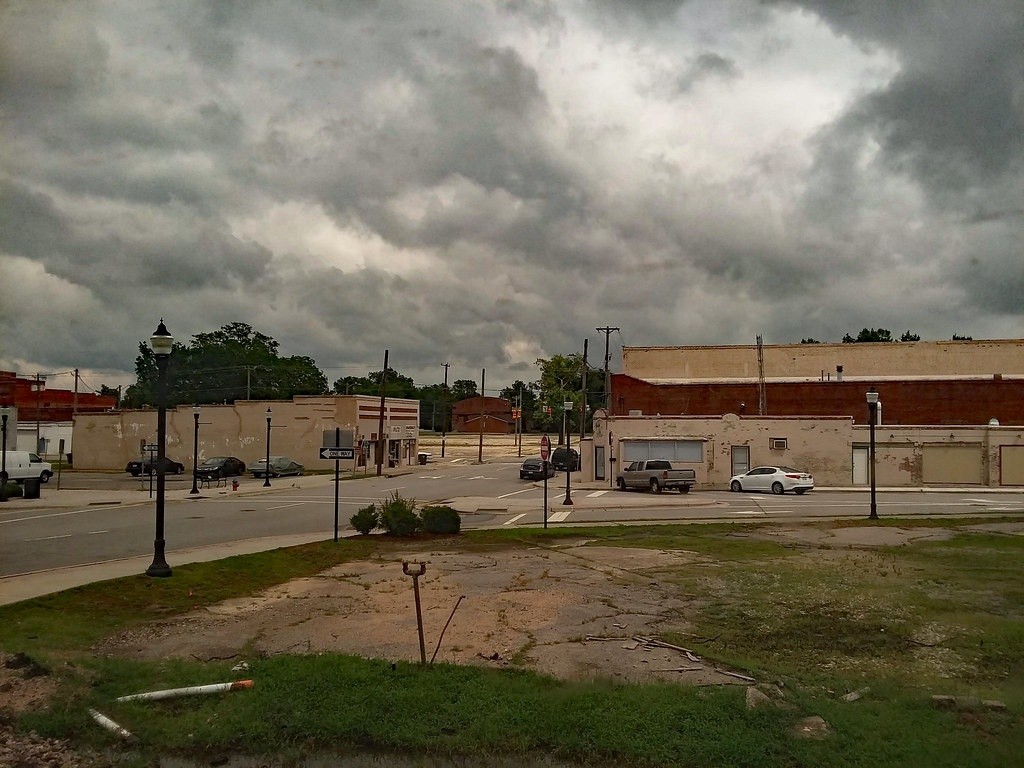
66 453 72 464
418 454 427 465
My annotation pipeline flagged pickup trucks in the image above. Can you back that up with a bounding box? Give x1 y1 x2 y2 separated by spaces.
615 459 697 494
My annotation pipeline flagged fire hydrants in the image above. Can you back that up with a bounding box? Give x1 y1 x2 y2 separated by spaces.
232 480 241 492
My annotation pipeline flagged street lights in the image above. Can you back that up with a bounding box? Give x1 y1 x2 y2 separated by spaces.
190 403 203 494
143 314 177 578
0 403 11 472
864 385 881 519
263 406 273 486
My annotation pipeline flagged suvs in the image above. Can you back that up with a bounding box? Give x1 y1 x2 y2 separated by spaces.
552 448 579 471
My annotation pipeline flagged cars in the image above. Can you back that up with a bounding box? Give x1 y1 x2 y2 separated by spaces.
520 458 556 478
125 456 185 477
192 457 246 478
247 456 305 479
727 466 814 494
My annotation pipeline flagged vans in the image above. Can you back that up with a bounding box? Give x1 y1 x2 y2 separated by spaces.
0 451 54 483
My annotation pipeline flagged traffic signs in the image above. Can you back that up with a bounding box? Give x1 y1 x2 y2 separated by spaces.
142 445 159 451
319 446 356 460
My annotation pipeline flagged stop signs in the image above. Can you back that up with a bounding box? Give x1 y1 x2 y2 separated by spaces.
539 436 549 460
